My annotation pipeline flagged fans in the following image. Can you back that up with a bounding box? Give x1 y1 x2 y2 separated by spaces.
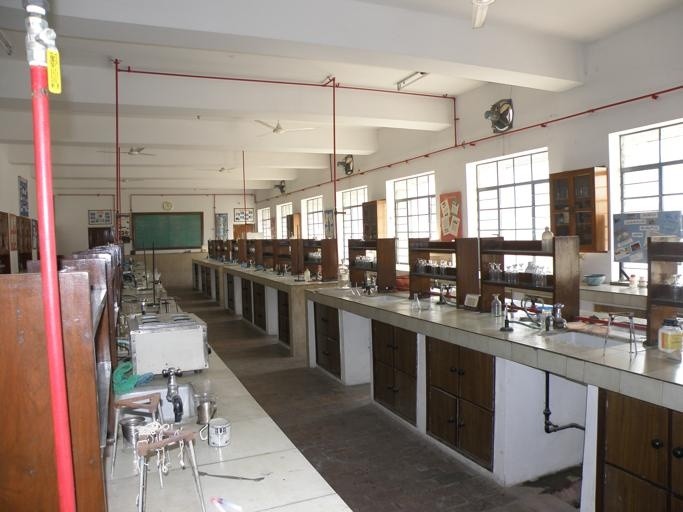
254 119 314 138
96 147 158 157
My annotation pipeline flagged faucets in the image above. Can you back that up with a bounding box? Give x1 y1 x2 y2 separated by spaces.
118 248 183 422
198 237 682 353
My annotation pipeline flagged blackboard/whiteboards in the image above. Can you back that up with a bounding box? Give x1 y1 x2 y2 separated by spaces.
131 212 204 251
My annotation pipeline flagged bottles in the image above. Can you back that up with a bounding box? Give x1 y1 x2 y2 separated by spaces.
410 293 421 316
630 274 637 294
542 226 555 255
657 317 682 353
491 293 502 318
305 268 311 282
414 257 457 275
488 261 547 288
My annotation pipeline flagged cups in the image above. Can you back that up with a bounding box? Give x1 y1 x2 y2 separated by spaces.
122 420 146 446
194 394 217 425
198 418 231 447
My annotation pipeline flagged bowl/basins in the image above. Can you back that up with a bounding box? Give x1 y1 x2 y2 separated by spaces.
585 273 606 286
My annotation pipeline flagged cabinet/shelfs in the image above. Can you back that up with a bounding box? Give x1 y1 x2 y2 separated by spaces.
548 166 608 253
0 244 123 511
286 213 300 239
190 236 682 511
361 199 388 240
